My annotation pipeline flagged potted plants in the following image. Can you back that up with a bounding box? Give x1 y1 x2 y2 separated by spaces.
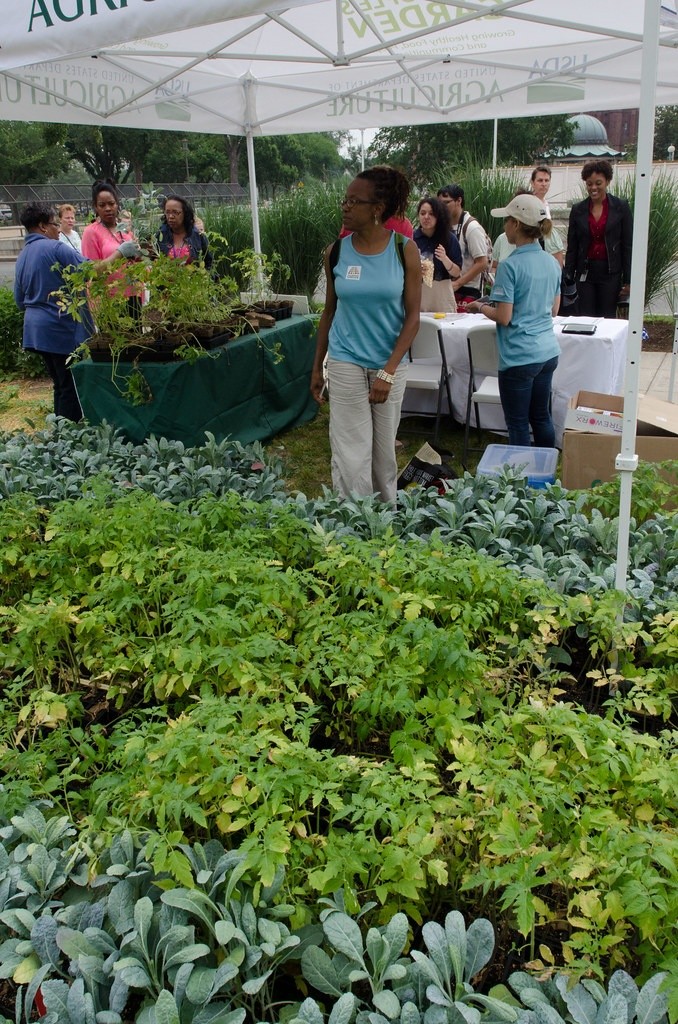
46 183 325 409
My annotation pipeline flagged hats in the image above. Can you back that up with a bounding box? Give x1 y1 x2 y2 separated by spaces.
490 193 548 228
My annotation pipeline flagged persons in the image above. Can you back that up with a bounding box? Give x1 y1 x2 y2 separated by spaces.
339 184 487 312
56 204 82 257
466 189 563 450
311 165 422 516
155 194 220 306
528 165 552 213
82 179 152 337
565 161 633 319
13 203 139 423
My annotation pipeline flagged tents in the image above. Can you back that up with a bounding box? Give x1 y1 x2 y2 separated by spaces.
0 0 678 597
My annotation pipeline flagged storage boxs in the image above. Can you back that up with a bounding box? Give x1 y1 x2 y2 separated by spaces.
562 390 678 520
476 442 560 489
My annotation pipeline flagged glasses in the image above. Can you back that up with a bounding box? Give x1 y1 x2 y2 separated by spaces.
341 199 380 208
164 211 184 215
443 198 456 204
49 222 61 228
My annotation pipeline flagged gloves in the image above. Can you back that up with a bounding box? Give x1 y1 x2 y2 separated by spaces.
116 240 140 258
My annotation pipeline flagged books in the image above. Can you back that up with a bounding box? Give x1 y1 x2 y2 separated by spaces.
561 316 604 325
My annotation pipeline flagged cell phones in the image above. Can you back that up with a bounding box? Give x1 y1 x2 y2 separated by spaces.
475 295 492 305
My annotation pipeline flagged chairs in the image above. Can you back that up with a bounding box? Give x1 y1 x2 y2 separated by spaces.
460 322 552 470
398 315 458 448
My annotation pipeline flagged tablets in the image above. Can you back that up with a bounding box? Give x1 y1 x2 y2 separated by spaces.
562 324 596 334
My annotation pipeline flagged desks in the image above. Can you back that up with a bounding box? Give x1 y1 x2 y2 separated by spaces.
70 314 319 451
400 312 628 447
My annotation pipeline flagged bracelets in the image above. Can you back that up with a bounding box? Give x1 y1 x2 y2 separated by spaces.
445 261 454 271
478 304 488 314
376 369 395 384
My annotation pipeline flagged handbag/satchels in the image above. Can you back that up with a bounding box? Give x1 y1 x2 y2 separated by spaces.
397 444 467 492
